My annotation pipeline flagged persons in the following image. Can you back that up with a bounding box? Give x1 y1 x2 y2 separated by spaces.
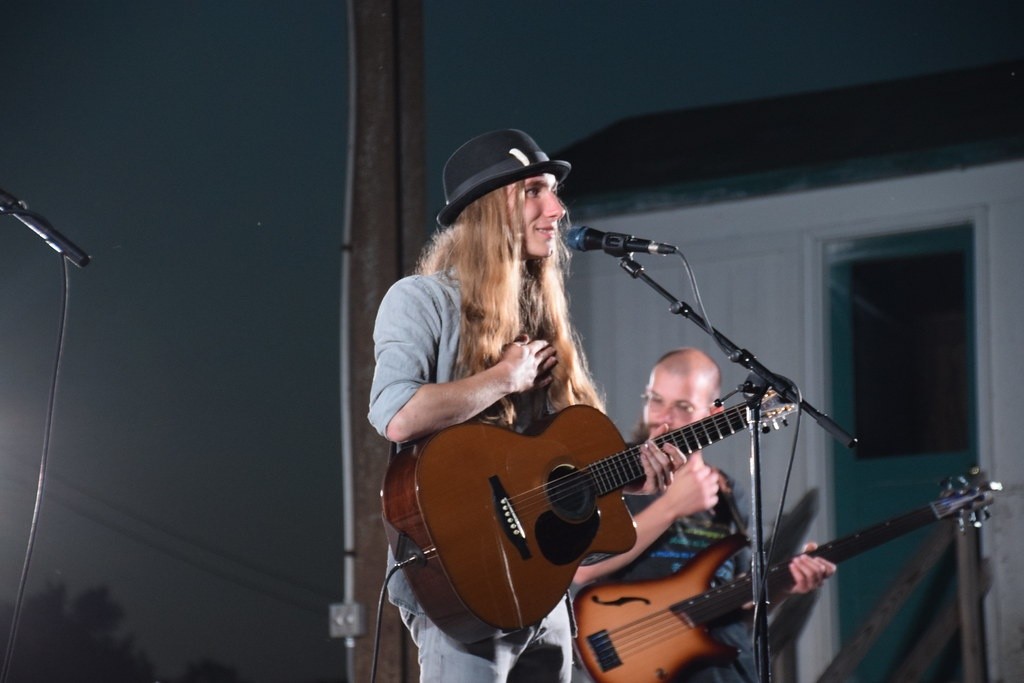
573 346 836 683
367 129 687 683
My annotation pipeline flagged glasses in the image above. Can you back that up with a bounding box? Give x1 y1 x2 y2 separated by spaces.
640 390 716 417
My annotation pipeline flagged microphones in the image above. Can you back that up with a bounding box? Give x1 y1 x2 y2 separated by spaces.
563 224 679 256
0 189 90 269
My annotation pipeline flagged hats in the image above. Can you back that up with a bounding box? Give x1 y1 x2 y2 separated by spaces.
436 129 571 227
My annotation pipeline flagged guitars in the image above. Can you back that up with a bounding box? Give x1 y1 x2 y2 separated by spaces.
571 468 995 683
379 384 799 649
